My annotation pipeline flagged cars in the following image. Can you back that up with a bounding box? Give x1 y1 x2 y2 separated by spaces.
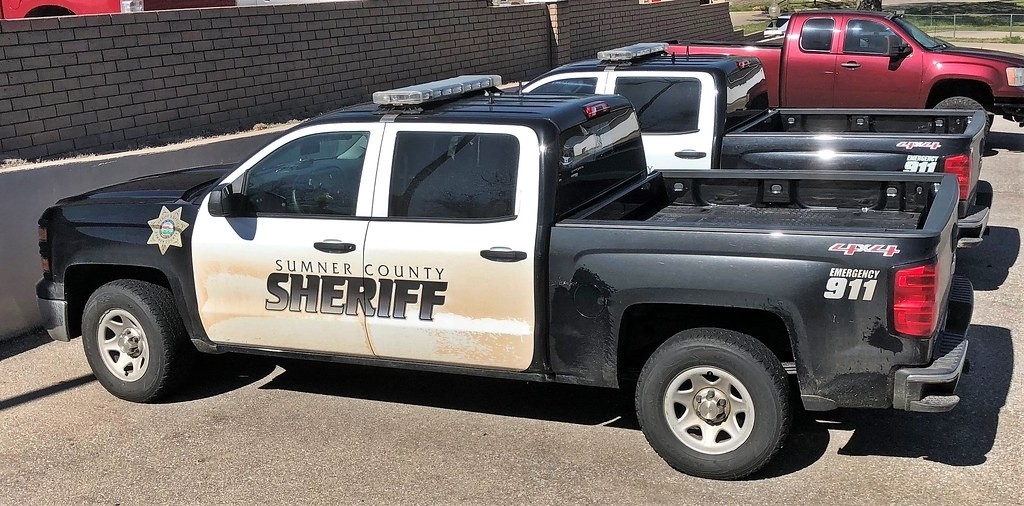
763 16 789 37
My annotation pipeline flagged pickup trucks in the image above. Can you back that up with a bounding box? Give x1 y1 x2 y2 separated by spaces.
662 11 1024 134
495 43 995 248
34 75 976 483
